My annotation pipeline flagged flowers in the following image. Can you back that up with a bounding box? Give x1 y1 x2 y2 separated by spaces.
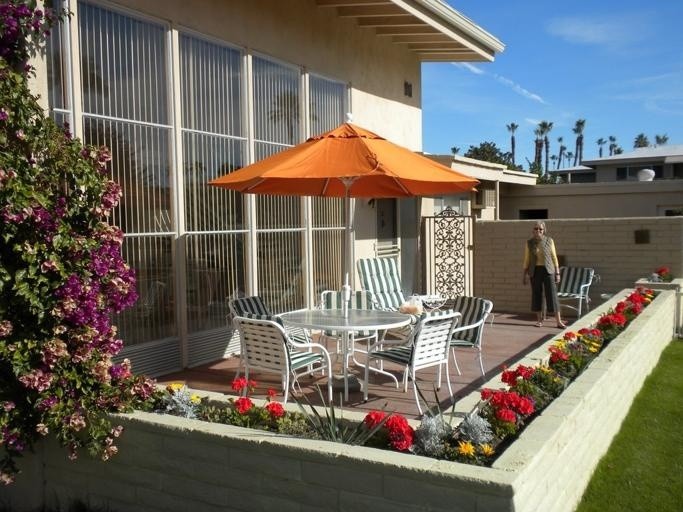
648 266 672 282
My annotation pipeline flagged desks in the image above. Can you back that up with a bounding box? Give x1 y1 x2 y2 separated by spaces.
279 306 412 406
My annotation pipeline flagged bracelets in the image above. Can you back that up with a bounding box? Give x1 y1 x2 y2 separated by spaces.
556 273 561 275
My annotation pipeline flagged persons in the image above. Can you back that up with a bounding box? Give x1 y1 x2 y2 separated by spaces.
207 123 480 375
522 222 566 329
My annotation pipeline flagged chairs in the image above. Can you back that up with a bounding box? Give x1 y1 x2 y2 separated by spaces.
403 297 494 391
228 296 314 378
542 266 595 320
233 316 333 407
319 290 379 370
357 257 414 344
364 312 462 419
